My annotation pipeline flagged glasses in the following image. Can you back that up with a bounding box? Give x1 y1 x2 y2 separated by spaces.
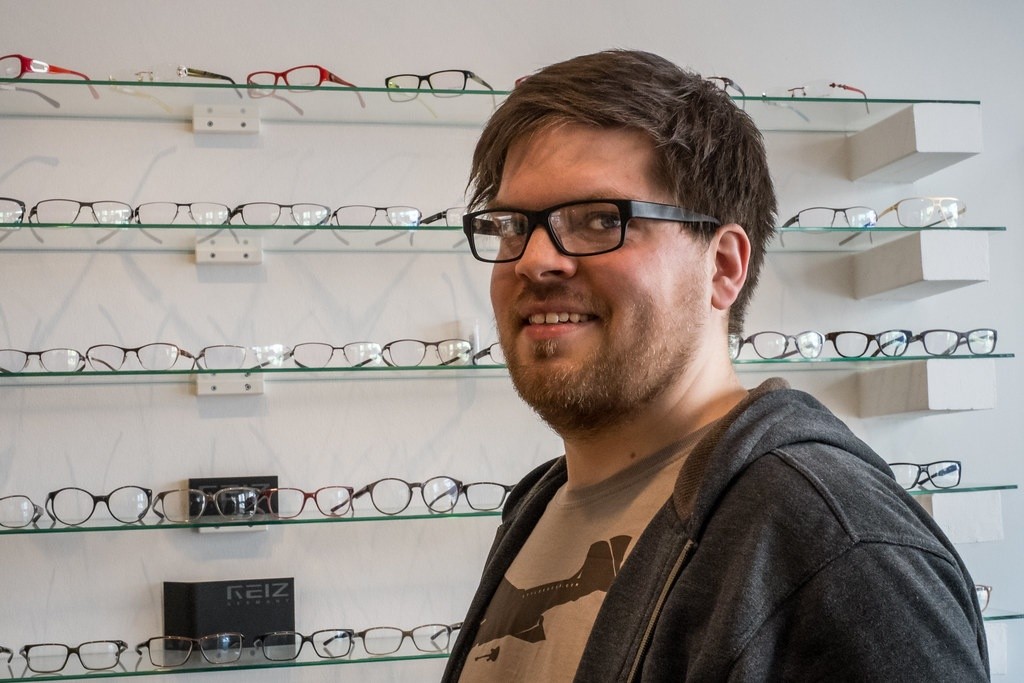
0 197 470 249
727 329 1001 362
710 75 871 120
461 199 720 263
776 198 968 244
0 341 507 372
0 476 518 528
888 459 964 490
973 585 994 614
0 621 468 672
3 54 503 113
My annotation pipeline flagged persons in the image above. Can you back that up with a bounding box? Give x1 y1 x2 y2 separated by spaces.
439 50 992 683
